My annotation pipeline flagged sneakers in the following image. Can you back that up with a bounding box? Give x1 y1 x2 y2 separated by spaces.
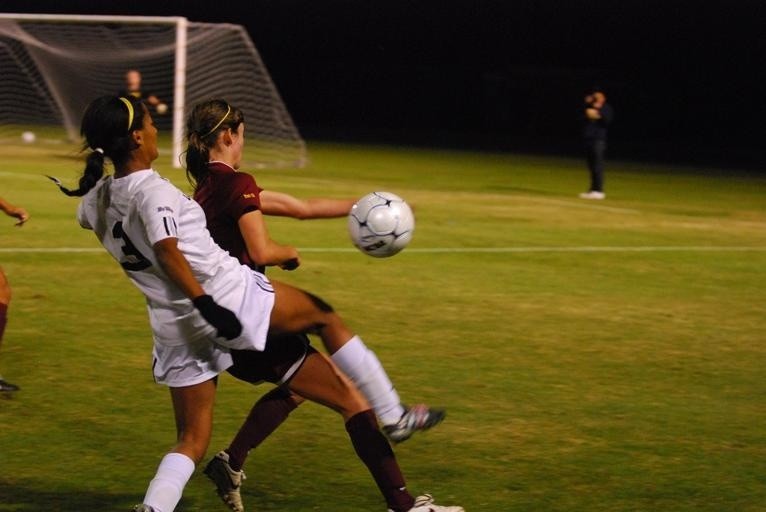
202 451 247 512
382 403 446 443
387 494 465 512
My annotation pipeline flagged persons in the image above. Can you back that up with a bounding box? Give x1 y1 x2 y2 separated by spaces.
42 93 448 511
580 91 615 200
117 69 169 115
0 198 30 392
183 98 462 510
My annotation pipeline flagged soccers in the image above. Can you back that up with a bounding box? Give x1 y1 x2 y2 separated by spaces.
156 103 169 115
348 190 415 257
21 132 35 145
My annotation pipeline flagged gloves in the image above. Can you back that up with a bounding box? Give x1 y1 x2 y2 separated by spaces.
193 294 243 341
